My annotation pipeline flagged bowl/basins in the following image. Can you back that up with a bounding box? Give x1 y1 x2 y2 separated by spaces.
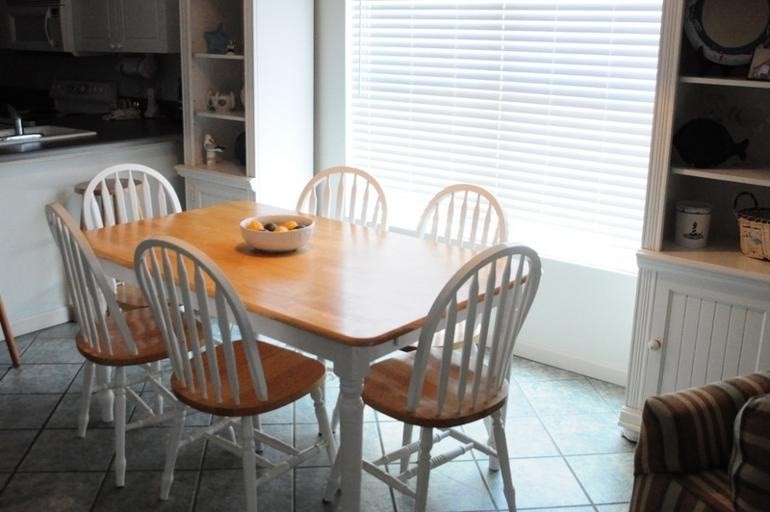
240 215 316 253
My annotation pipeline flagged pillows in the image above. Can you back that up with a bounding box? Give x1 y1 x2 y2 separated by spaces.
729 394 770 512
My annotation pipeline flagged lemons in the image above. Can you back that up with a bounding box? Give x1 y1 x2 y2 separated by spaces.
247 219 310 232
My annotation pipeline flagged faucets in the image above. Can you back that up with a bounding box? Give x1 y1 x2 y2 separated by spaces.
0 101 44 143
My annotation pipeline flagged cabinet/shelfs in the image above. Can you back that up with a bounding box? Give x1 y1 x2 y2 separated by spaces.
617 0 770 444
174 0 313 210
65 0 179 54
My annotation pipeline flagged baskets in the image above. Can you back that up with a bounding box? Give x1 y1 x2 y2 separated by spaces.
733 192 770 260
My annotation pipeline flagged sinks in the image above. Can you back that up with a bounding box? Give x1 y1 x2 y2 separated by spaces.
0 124 98 150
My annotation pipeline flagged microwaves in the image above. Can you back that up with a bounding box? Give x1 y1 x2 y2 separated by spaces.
0 0 73 52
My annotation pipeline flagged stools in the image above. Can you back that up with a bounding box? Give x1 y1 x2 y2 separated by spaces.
75 178 144 321
0 295 21 368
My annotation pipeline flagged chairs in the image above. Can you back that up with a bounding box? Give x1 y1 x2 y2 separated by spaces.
295 166 388 435
134 235 341 512
629 368 770 512
330 184 509 435
320 242 542 512
45 202 238 488
82 163 182 292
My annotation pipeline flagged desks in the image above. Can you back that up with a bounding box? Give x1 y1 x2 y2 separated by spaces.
82 201 542 512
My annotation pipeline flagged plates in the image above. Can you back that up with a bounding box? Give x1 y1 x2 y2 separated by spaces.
684 0 769 66
